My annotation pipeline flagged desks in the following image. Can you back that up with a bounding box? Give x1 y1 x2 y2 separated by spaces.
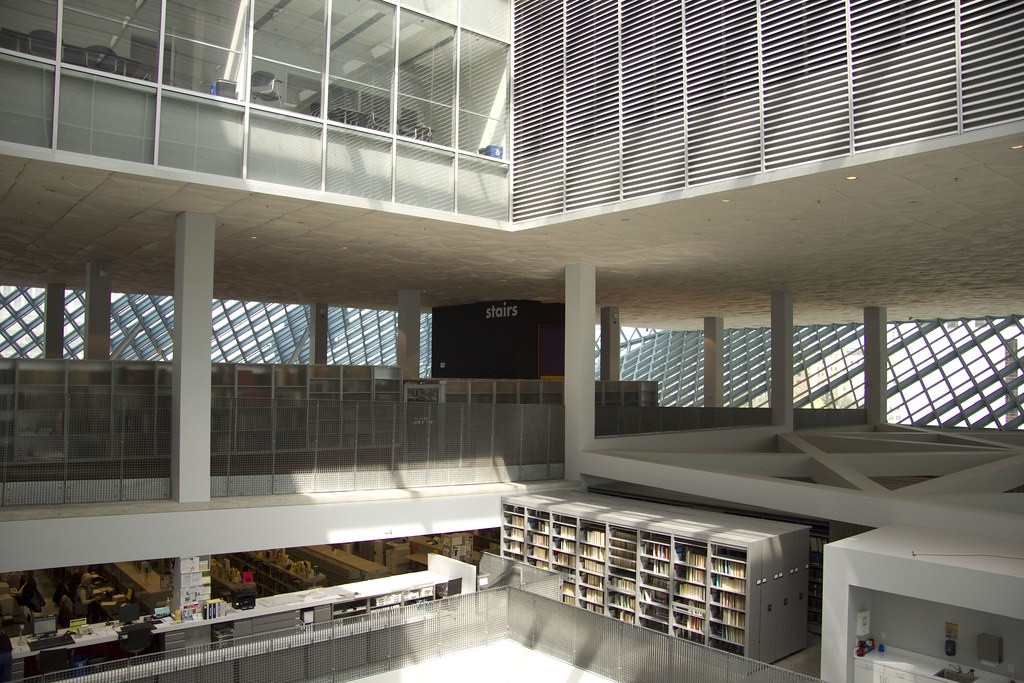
328 107 389 132
0 27 157 82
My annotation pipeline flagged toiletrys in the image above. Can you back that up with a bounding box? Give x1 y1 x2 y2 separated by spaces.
878 632 886 654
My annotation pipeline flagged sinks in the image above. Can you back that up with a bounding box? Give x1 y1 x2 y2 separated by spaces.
932 667 979 683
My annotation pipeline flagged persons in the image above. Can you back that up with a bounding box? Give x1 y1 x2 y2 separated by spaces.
73 565 88 589
73 572 102 623
0 610 13 683
10 571 46 611
53 573 76 605
58 595 79 627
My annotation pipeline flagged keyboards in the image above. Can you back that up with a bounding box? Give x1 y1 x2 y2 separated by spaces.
27 634 76 652
121 622 158 633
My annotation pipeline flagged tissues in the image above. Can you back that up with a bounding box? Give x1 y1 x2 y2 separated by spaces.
976 632 1003 668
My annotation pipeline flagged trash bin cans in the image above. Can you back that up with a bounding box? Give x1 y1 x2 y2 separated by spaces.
68 654 106 677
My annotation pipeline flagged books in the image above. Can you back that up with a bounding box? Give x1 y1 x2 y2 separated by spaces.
510 515 746 655
176 598 226 622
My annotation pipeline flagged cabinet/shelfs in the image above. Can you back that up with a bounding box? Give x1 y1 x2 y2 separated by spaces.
500 489 813 677
854 660 945 683
0 357 852 683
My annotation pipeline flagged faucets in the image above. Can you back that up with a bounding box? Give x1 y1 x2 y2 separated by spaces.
948 664 963 673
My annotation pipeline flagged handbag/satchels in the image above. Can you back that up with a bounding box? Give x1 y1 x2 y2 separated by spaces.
22 582 45 612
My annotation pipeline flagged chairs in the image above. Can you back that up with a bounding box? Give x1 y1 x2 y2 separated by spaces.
118 626 155 659
22 29 436 143
35 646 75 680
58 596 80 628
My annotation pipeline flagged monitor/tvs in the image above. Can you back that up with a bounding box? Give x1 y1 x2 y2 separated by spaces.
33 614 57 640
119 604 140 625
